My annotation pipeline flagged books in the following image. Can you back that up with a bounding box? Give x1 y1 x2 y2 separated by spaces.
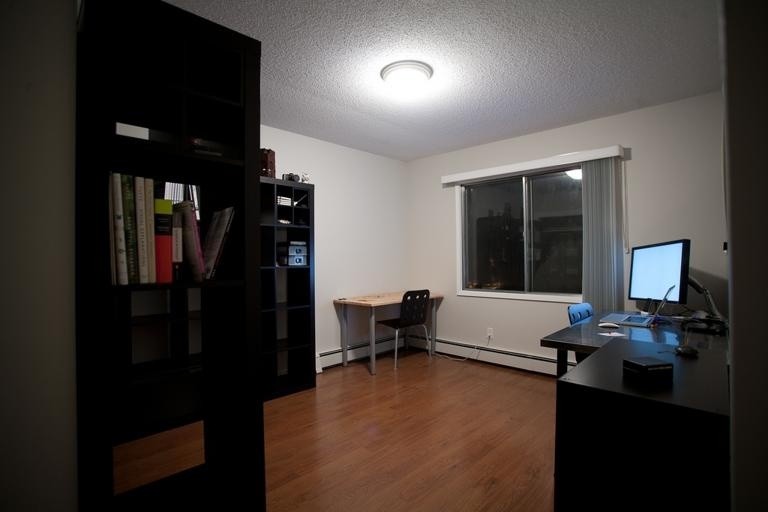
108 170 241 287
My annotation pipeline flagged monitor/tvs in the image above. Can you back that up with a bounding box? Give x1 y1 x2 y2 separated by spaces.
628 239 690 305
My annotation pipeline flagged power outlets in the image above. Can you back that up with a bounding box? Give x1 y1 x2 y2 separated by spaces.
486 328 494 337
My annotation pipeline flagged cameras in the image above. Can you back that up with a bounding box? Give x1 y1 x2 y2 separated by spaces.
282 173 300 182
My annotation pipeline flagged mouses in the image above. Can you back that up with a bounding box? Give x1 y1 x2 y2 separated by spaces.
673 346 698 359
598 322 619 327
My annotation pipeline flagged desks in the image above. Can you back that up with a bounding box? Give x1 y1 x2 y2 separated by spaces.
333 292 443 375
540 312 730 378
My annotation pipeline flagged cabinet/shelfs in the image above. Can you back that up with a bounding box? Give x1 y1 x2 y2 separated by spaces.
260 177 316 403
554 337 731 512
75 1 266 511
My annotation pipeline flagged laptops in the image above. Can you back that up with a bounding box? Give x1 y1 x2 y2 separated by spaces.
599 285 676 327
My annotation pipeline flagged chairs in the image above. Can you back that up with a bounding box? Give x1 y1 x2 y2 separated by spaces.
376 289 432 369
568 303 594 364
